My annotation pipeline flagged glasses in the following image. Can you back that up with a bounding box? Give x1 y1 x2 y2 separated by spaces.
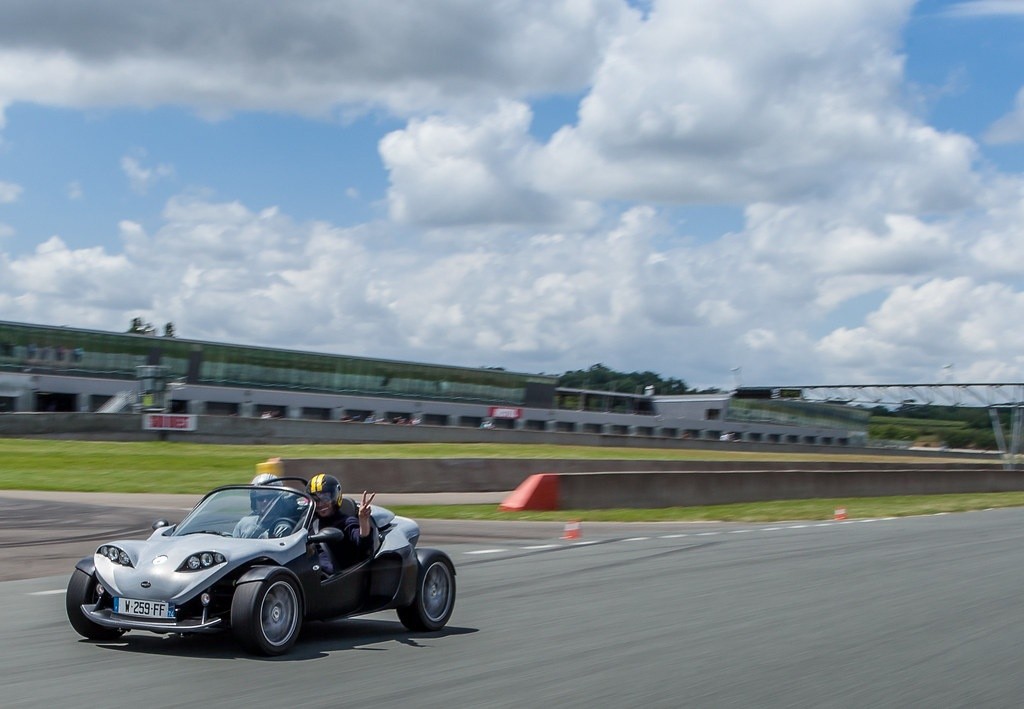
253 494 278 503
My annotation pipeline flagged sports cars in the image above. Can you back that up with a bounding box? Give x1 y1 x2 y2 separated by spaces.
67 472 456 654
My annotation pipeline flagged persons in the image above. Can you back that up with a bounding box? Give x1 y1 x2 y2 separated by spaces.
228 407 278 420
295 471 378 572
478 413 498 431
231 473 299 538
680 431 743 443
340 414 423 426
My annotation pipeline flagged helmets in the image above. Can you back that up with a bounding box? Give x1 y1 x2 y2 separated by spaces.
249 473 284 510
306 473 343 511
